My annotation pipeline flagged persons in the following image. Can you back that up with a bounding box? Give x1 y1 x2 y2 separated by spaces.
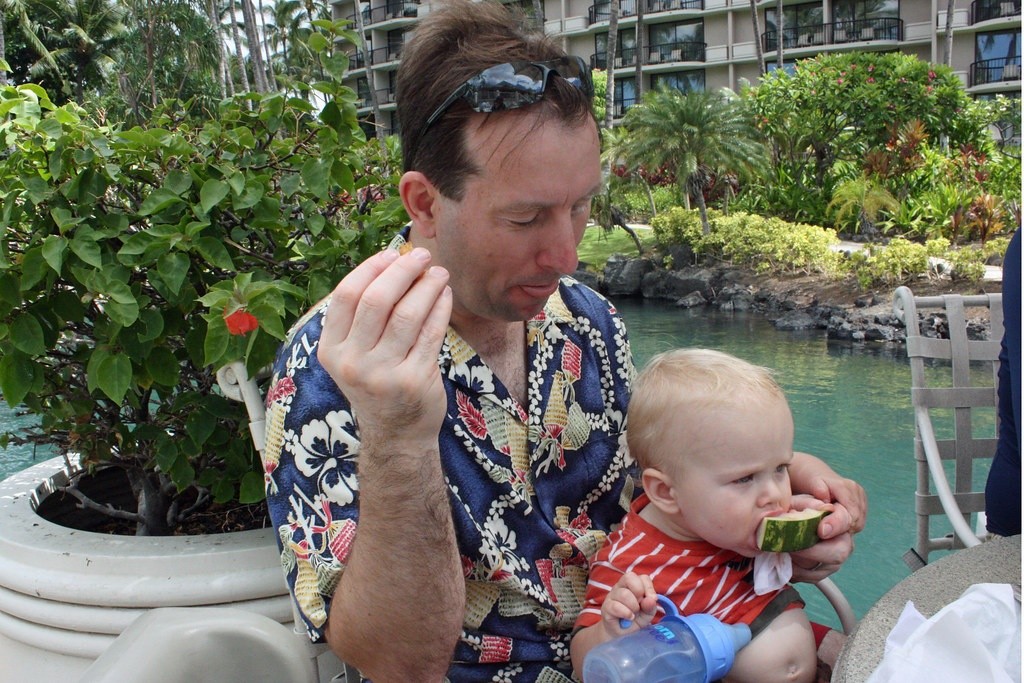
985 224 1021 538
569 348 867 683
265 7 864 682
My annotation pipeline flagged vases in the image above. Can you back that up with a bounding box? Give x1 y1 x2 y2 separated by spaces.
0 450 365 683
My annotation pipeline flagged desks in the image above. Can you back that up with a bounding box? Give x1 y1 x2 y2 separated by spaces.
829 532 1021 683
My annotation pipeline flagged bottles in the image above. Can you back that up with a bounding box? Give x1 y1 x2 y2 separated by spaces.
582 594 752 683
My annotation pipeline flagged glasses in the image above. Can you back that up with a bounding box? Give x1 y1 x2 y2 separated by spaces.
404 56 593 174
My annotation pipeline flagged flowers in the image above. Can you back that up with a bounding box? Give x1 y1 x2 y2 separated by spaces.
0 17 414 536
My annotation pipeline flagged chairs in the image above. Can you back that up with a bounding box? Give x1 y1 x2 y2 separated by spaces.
216 357 857 683
893 285 1004 573
798 26 875 46
617 0 681 18
614 48 682 69
385 9 404 21
388 93 396 102
386 52 396 61
1000 2 1015 16
1001 63 1021 80
